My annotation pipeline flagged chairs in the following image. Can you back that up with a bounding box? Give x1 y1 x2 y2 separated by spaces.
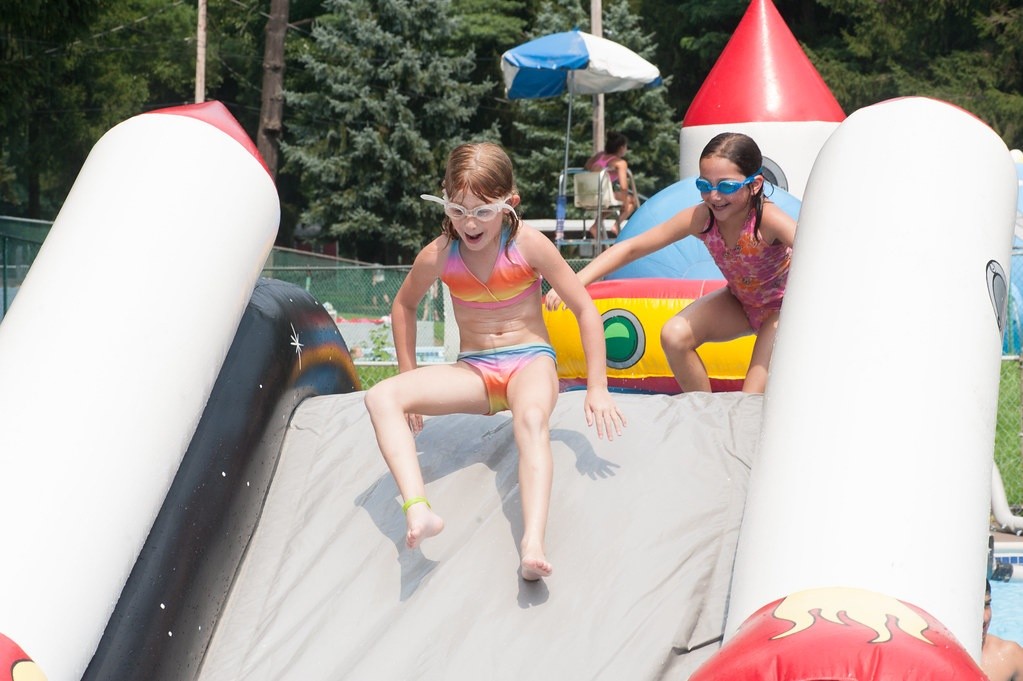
572 169 626 240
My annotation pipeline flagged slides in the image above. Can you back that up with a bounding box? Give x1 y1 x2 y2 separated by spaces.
0 93 1018 681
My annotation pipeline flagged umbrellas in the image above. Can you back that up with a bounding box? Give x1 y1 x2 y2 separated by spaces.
500 27 662 239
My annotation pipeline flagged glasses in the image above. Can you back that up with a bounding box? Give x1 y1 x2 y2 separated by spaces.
695 173 758 194
441 189 512 222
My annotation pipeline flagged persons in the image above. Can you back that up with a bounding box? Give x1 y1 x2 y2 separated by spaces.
583 135 637 240
545 133 798 394
981 580 1023 681
364 143 628 580
